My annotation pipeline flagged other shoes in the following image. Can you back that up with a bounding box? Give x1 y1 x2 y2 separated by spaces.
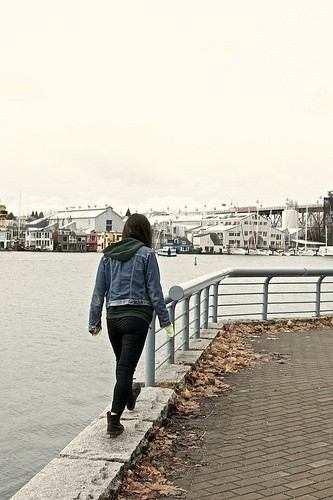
106 411 124 438
127 383 141 410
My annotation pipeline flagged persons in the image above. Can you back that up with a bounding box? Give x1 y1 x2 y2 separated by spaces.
88 213 175 437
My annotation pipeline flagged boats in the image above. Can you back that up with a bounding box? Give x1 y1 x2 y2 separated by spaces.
223 245 319 256
158 246 177 256
